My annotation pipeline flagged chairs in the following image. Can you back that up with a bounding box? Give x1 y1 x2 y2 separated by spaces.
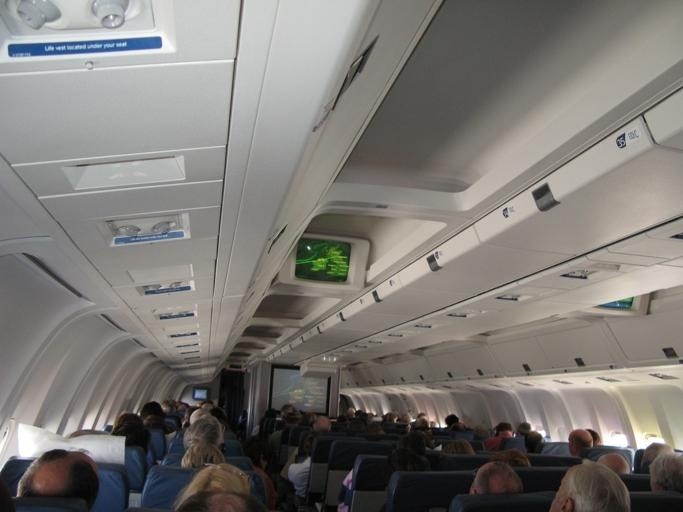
0 456 129 511
437 453 492 474
627 491 683 511
527 455 583 467
449 492 556 512
68 431 145 493
162 414 252 468
619 473 655 491
294 436 335 508
106 398 183 458
257 412 289 477
347 409 484 454
510 466 572 493
381 470 475 512
140 467 258 512
342 454 391 511
289 425 310 465
313 440 390 512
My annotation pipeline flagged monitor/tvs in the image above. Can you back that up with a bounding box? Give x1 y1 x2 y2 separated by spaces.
581 294 651 316
277 234 370 292
190 385 210 402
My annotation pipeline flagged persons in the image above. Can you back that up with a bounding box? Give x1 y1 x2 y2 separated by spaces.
587 429 600 446
189 408 210 424
516 422 531 439
279 430 324 506
112 413 143 432
336 431 433 512
640 443 673 476
175 489 268 512
490 449 531 468
568 429 593 457
413 412 466 432
596 453 630 477
485 422 512 451
648 452 683 496
16 449 99 509
470 461 522 494
439 439 476 457
175 463 249 510
111 424 151 456
144 416 163 431
549 462 632 512
180 444 224 469
182 416 223 451
524 430 544 454
313 416 332 433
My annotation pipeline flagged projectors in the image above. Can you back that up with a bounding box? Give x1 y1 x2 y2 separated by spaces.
299 360 337 380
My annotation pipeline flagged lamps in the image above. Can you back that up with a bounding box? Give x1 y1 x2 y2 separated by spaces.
91 0 130 29
499 437 531 454
152 221 176 234
116 225 140 236
16 0 62 29
